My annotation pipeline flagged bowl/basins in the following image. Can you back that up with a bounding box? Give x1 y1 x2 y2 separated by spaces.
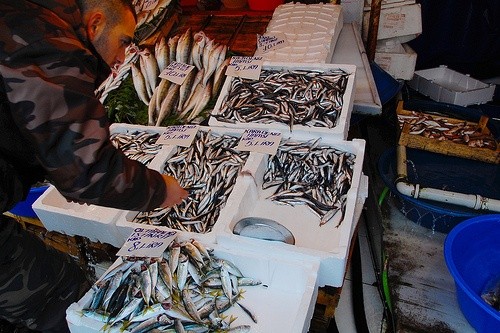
6 185 50 217
443 213 500 333
379 104 500 233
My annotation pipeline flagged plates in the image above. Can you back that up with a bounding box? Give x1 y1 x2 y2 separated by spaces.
232 217 296 249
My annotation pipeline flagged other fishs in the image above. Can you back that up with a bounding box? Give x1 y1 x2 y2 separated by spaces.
209 68 354 133
81 238 262 333
397 111 496 152
96 28 229 125
262 135 357 227
106 130 251 234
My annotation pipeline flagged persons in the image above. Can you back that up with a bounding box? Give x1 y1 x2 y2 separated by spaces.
0 0 189 332
